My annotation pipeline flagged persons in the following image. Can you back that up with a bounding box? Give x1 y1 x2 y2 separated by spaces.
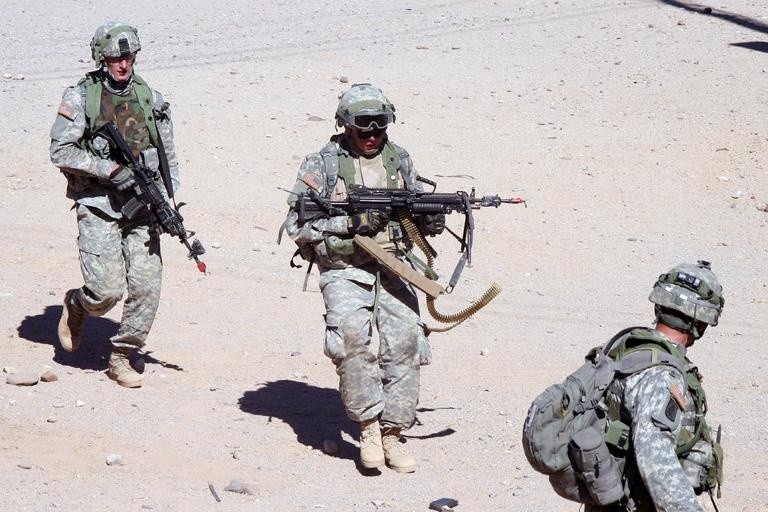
583 260 724 512
282 83 446 475
48 21 179 388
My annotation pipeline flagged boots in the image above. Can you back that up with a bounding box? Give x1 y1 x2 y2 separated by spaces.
356 414 385 469
379 426 418 474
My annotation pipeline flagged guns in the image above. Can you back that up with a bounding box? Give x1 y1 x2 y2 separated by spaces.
93 120 210 274
300 187 526 264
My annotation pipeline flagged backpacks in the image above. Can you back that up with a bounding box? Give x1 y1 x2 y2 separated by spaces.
520 325 705 505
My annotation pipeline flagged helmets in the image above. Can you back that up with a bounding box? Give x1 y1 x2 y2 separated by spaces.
90 21 140 61
337 81 394 133
649 256 725 332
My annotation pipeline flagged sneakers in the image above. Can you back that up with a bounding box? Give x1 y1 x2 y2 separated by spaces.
56 288 91 353
107 344 145 389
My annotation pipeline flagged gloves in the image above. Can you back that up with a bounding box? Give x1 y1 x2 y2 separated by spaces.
350 209 389 234
110 167 136 191
424 213 446 234
